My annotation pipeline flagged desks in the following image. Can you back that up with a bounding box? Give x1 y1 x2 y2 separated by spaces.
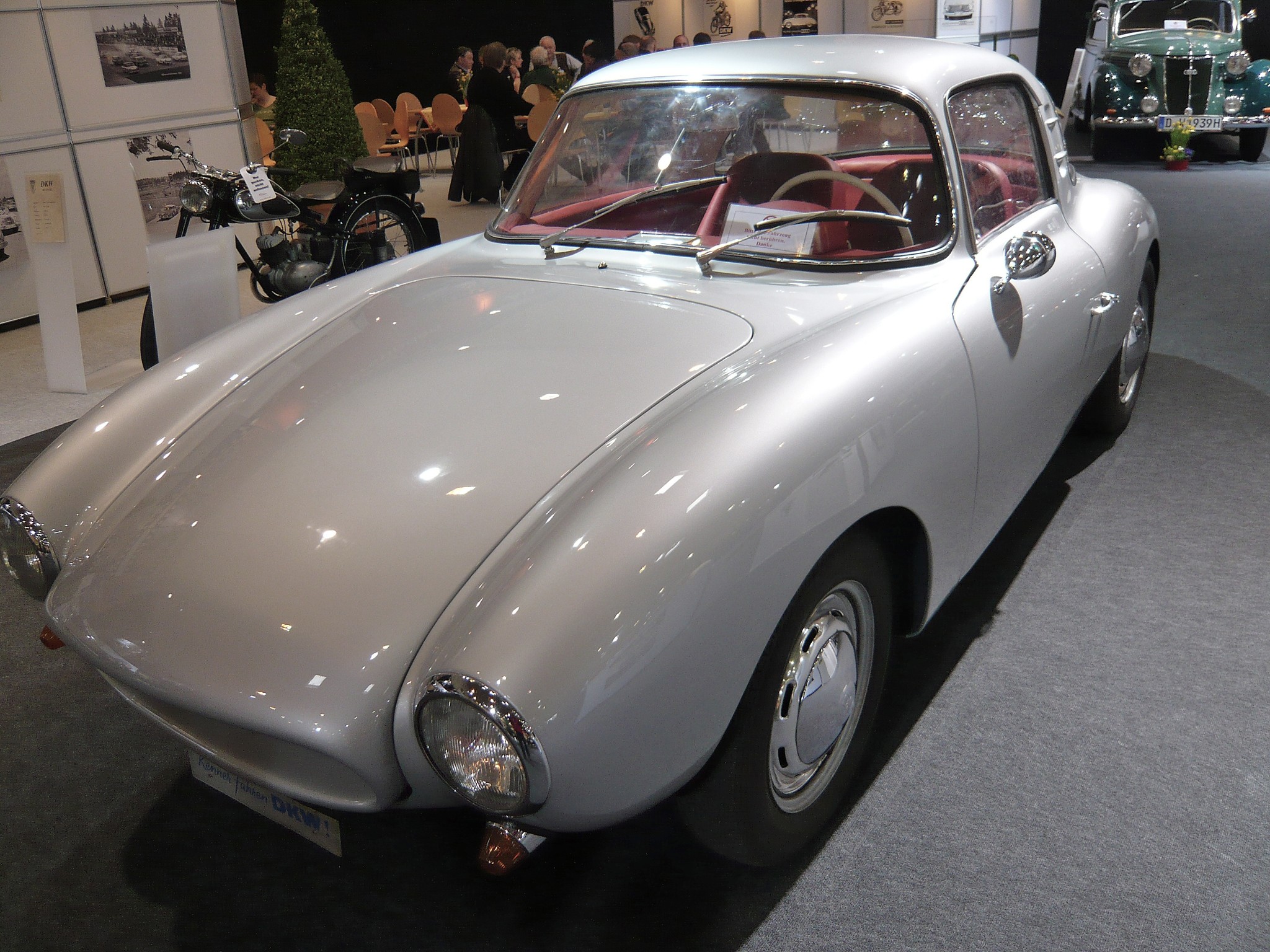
408 99 468 170
516 110 619 184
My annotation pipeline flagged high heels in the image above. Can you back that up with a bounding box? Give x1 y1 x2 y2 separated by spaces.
503 183 512 193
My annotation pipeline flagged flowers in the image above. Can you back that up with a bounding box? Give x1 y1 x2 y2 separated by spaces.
455 67 474 98
1159 115 1196 162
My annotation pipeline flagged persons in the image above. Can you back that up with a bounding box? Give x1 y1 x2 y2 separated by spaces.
446 31 765 203
249 74 277 137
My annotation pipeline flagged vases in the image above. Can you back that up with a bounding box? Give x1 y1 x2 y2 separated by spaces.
1166 159 1190 171
463 97 468 107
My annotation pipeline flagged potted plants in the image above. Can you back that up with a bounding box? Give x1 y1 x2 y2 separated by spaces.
272 0 377 232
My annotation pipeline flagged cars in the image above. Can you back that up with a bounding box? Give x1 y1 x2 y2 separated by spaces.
98 46 186 76
0 36 1164 877
1070 0 1270 163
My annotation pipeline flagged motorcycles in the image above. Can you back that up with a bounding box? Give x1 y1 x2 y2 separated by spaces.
139 128 442 370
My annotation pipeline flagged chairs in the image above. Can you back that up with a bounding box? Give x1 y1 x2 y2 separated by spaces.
396 89 434 173
429 93 462 178
356 111 386 156
698 150 845 237
376 99 426 193
526 100 594 198
850 155 1008 242
369 98 435 172
353 102 378 116
522 84 557 104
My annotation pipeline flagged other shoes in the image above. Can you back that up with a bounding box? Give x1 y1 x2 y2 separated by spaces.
584 163 608 186
621 157 657 182
